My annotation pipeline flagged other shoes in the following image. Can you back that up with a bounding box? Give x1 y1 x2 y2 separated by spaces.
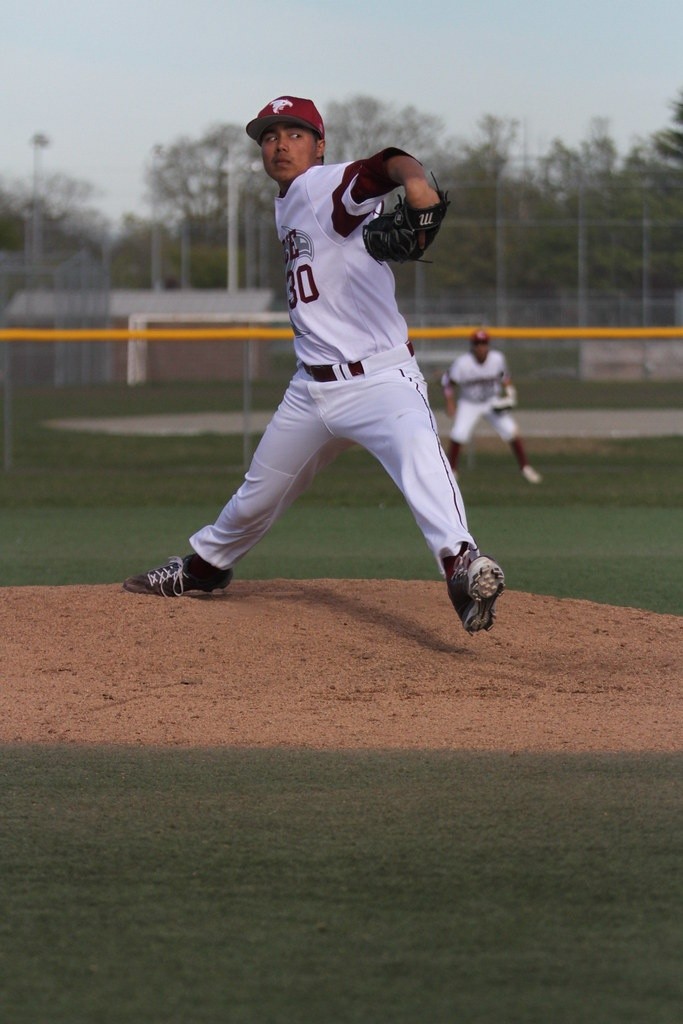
521 464 541 485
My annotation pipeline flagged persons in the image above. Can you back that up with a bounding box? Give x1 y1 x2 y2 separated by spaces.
122 97 505 636
441 330 542 484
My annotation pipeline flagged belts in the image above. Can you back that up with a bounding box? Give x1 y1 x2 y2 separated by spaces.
301 342 414 382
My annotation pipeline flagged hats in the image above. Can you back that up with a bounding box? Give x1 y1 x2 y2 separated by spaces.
246 96 325 147
471 331 489 343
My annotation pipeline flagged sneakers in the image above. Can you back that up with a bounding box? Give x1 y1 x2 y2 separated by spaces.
446 542 505 637
123 553 233 598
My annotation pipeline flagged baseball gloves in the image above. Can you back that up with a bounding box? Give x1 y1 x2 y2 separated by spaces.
491 385 518 416
362 189 449 264
